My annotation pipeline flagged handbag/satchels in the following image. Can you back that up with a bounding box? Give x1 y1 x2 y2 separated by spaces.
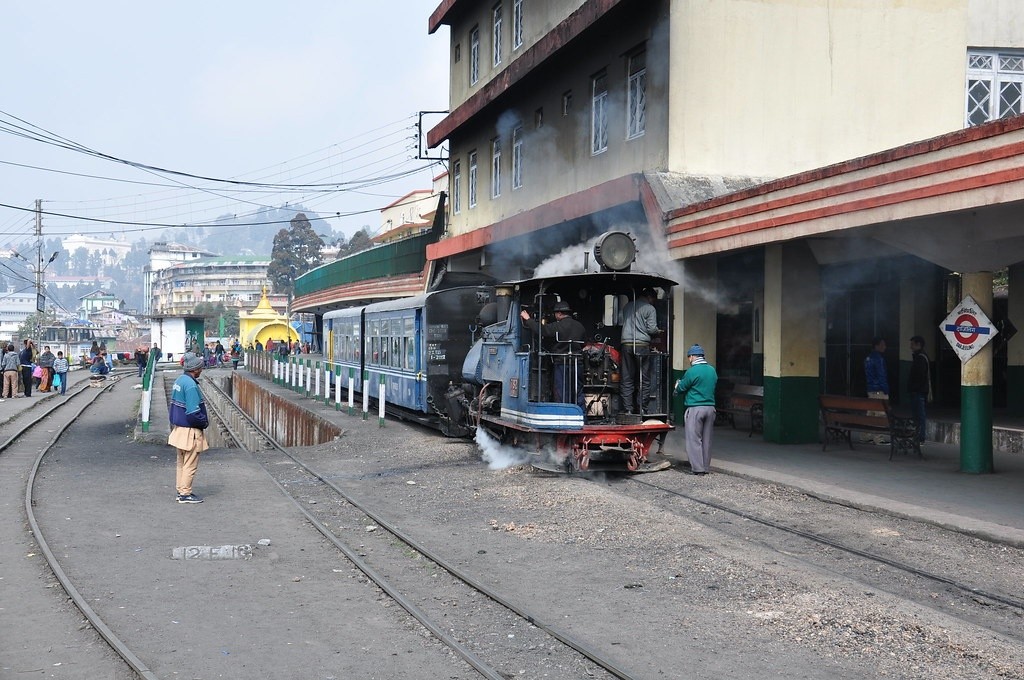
54 373 61 387
926 390 934 403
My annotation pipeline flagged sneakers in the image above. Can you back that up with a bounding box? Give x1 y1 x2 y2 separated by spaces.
178 493 204 503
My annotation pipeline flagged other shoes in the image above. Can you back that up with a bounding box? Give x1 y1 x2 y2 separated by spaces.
876 439 891 445
860 438 873 443
1 387 66 398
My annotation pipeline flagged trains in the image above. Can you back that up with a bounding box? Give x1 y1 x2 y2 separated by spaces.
322 229 682 470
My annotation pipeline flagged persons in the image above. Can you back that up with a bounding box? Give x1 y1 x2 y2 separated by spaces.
90 341 106 360
186 337 242 370
168 351 208 503
858 338 892 445
906 335 931 445
134 343 161 377
675 343 718 475
617 287 660 414
248 337 315 364
0 338 69 399
521 301 588 424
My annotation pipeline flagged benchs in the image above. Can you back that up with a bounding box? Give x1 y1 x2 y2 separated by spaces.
815 391 928 461
715 384 764 438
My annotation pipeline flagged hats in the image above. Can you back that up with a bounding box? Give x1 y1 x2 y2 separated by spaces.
57 351 63 355
687 343 705 356
553 301 572 312
180 352 204 372
